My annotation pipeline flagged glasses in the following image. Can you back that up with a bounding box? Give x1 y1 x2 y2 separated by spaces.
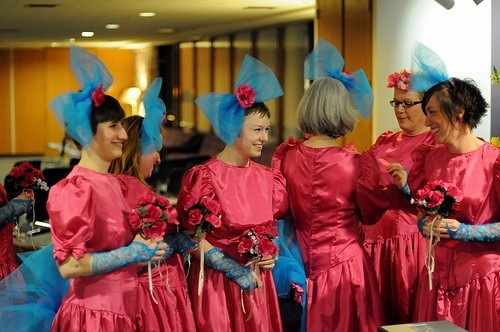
390 100 423 108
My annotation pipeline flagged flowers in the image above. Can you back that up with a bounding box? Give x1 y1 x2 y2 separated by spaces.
128 189 179 241
411 179 463 221
386 69 413 93
10 162 49 192
344 70 355 87
490 66 500 88
160 111 168 125
491 136 500 148
184 196 222 239
290 282 304 304
234 84 255 108
91 84 106 107
238 225 280 259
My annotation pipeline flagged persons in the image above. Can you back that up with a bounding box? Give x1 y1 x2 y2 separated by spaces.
0 182 35 332
359 73 446 332
407 78 500 332
271 79 395 332
176 102 288 332
108 115 198 332
46 90 167 332
